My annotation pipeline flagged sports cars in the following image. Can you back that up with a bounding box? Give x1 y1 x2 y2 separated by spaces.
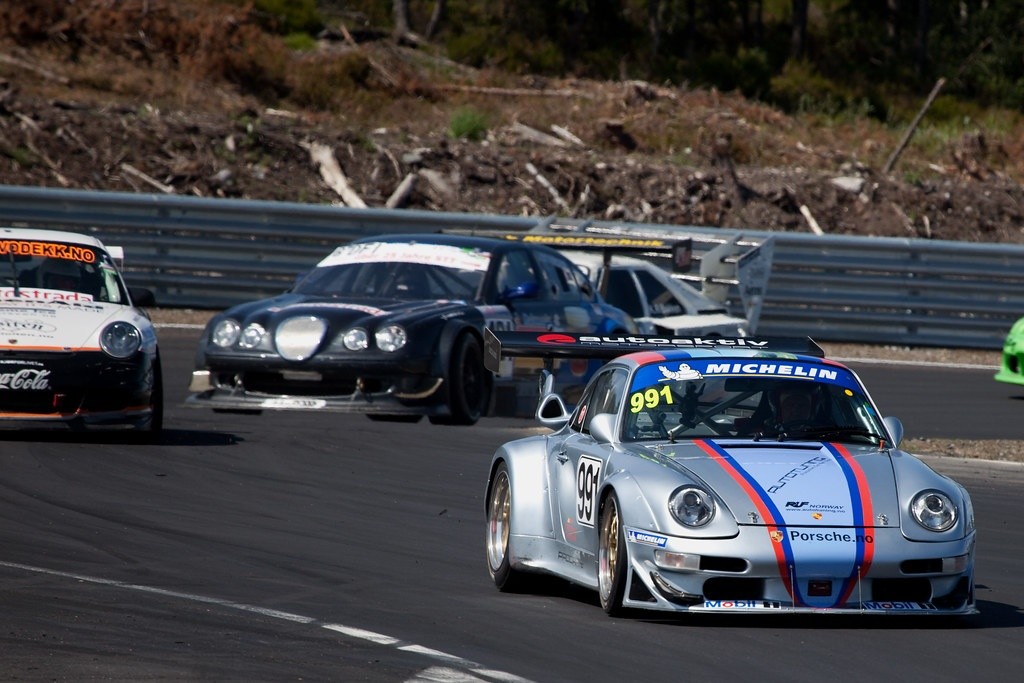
1 224 166 445
184 226 676 428
476 324 986 625
521 216 776 339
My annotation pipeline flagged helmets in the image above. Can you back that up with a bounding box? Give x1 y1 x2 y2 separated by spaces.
767 380 811 422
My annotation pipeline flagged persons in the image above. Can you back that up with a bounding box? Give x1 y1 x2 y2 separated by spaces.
762 385 829 444
659 238 701 276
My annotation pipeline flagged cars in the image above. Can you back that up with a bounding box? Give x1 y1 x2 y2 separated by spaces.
993 315 1024 388
